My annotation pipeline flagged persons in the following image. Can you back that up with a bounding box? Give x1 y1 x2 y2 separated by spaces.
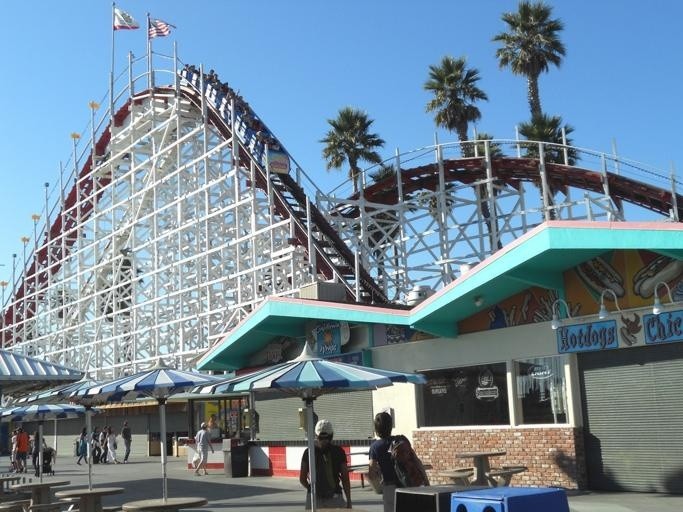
194 421 214 474
7 419 130 472
299 418 351 510
183 64 280 151
368 411 430 512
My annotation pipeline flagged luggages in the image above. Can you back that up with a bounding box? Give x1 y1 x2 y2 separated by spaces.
41 452 54 476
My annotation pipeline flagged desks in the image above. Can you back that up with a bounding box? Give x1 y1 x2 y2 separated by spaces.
346 450 506 488
0 477 208 512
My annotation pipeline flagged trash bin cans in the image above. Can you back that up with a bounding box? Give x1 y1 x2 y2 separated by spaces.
394 485 569 512
222 438 249 478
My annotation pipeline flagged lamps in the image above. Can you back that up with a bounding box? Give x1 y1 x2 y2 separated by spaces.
549 282 682 330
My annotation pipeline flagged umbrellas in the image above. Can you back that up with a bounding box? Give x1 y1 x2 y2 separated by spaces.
0 348 84 404
192 341 427 512
0 403 107 482
11 370 102 490
67 356 235 501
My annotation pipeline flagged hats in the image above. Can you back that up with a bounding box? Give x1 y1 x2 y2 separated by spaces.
315 420 333 437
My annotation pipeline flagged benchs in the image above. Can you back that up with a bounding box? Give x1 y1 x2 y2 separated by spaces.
485 465 528 487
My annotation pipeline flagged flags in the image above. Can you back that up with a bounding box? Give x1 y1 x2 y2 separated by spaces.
112 7 139 30
147 18 176 40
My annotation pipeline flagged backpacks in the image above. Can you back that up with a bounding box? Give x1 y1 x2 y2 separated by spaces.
388 434 428 487
316 445 338 492
